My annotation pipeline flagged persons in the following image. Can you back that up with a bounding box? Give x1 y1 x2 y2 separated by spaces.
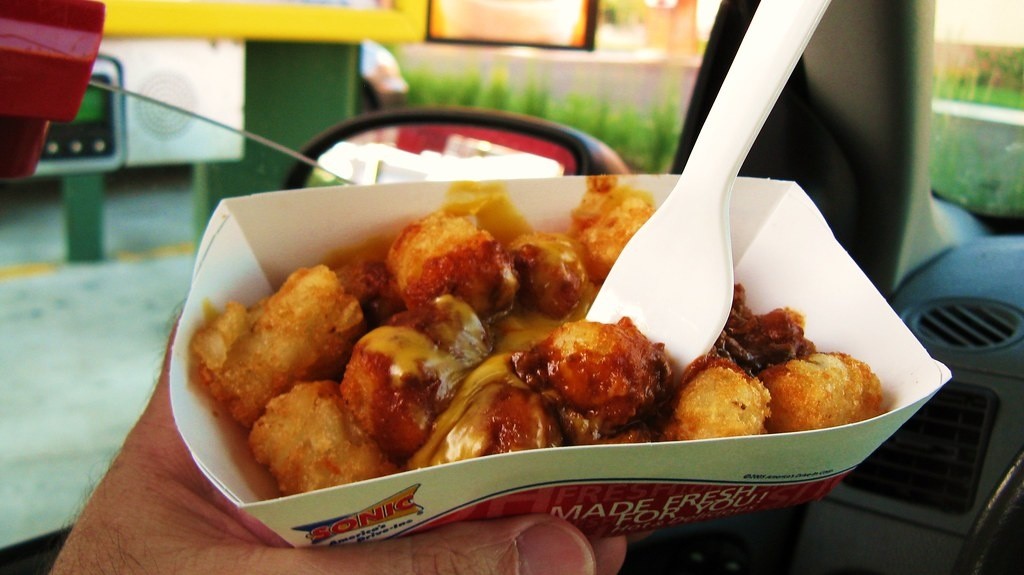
48 304 627 575
